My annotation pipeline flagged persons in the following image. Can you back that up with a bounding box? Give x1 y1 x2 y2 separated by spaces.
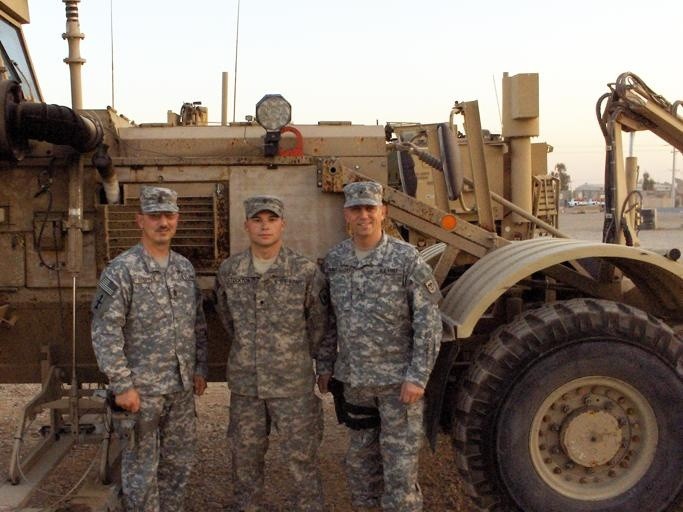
89 187 211 512
211 196 330 511
315 181 444 511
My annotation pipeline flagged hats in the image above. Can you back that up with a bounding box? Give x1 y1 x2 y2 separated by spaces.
343 182 383 208
138 186 180 214
243 196 283 220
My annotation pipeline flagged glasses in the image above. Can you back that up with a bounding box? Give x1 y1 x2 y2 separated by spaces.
146 212 176 221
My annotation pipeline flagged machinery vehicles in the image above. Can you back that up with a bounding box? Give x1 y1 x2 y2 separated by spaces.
0 0 683 512
